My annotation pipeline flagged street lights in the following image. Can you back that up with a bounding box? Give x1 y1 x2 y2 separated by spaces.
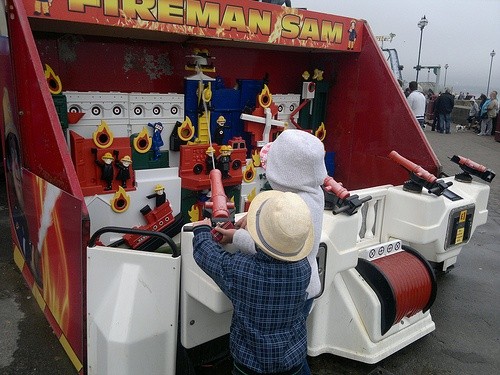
416 14 428 90
486 50 495 97
444 64 449 86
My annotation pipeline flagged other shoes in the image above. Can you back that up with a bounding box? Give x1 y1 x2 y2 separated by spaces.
437 130 439 132
440 130 444 133
467 127 470 130
445 131 451 134
432 129 435 131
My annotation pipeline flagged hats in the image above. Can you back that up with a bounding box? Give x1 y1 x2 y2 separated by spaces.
247 190 314 263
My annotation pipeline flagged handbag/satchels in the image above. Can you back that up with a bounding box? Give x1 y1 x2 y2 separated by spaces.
481 112 489 119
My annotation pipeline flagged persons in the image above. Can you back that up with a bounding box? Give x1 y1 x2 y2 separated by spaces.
405 81 426 130
458 91 499 136
404 88 410 99
193 190 315 375
215 129 327 322
421 89 455 134
